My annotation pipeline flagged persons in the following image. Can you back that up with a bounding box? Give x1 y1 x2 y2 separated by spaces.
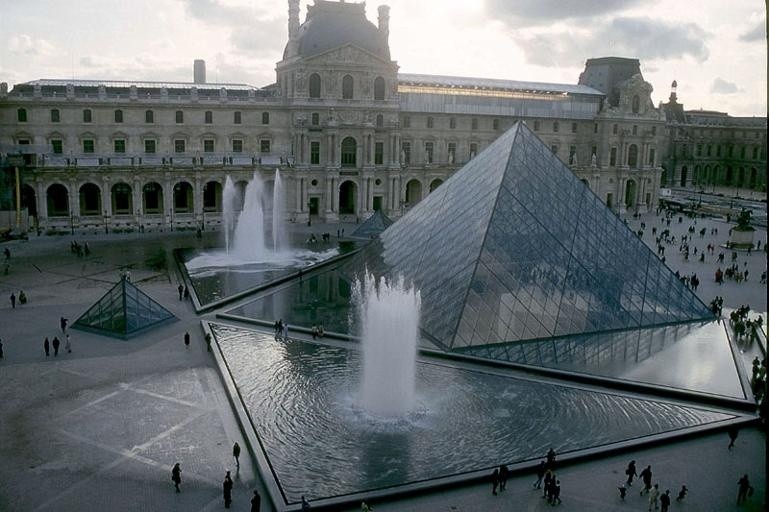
533 448 562 506
184 286 188 300
2 248 11 263
312 323 318 340
319 322 325 338
9 290 29 310
0 339 4 358
615 197 769 428
491 468 498 496
223 476 232 509
184 331 190 352
43 316 74 358
178 284 183 301
736 474 753 505
728 424 741 451
361 500 369 512
616 460 671 512
71 241 92 257
499 464 509 491
302 227 344 245
676 485 688 502
251 490 260 512
273 318 288 337
225 471 233 502
302 496 308 512
172 464 182 493
206 333 211 352
